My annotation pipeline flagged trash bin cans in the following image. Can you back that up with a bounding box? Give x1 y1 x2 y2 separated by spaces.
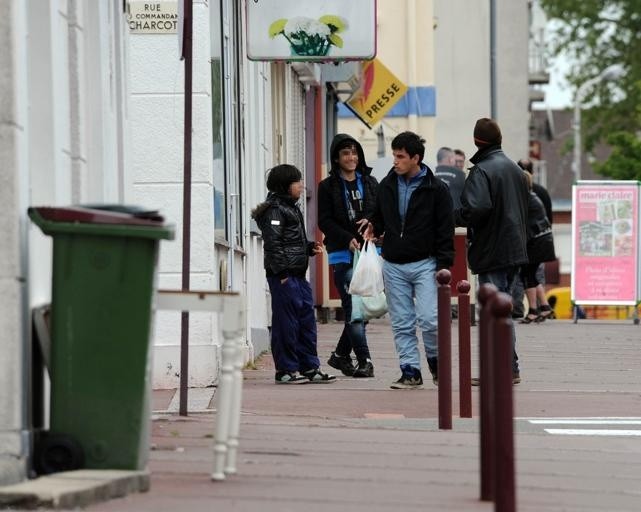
28 204 175 474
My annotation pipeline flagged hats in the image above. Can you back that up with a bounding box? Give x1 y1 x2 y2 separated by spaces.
474 118 501 147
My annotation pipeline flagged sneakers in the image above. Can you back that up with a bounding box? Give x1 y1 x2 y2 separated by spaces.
471 372 520 386
427 357 438 385
328 352 356 376
275 371 310 384
303 369 336 382
353 362 374 377
390 365 424 389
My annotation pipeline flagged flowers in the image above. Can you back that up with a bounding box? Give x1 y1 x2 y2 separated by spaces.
268 14 346 56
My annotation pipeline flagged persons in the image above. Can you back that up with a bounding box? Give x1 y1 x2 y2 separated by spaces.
356 131 455 390
455 148 465 169
518 168 556 324
434 146 468 224
511 158 546 316
253 165 337 385
319 132 379 376
459 116 530 388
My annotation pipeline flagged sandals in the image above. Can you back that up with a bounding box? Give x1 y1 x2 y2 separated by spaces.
519 305 555 324
